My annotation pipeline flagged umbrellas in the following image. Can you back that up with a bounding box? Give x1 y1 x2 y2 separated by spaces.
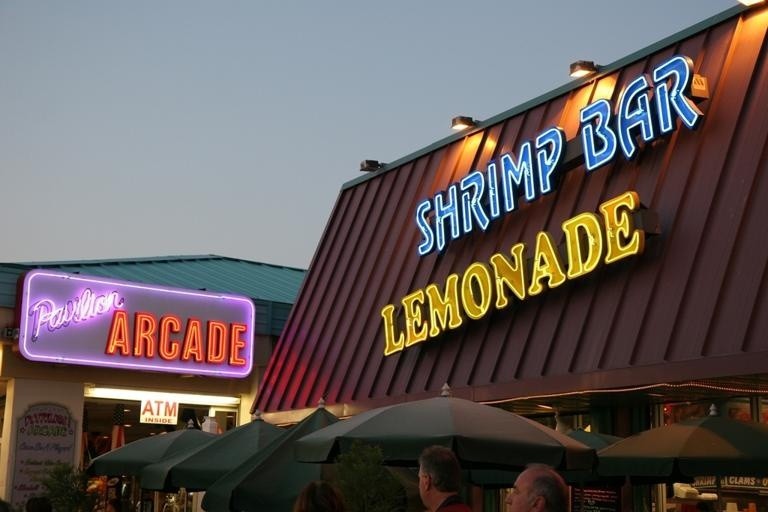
202 397 356 510
296 378 590 483
140 408 289 492
86 418 220 477
557 422 632 512
596 403 768 511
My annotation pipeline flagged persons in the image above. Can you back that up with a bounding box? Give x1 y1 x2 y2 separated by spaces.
500 460 573 511
107 497 128 512
292 478 347 511
26 496 53 511
415 443 473 512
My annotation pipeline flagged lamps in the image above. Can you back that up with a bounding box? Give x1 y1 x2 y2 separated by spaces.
569 60 603 77
360 160 388 172
451 116 479 130
